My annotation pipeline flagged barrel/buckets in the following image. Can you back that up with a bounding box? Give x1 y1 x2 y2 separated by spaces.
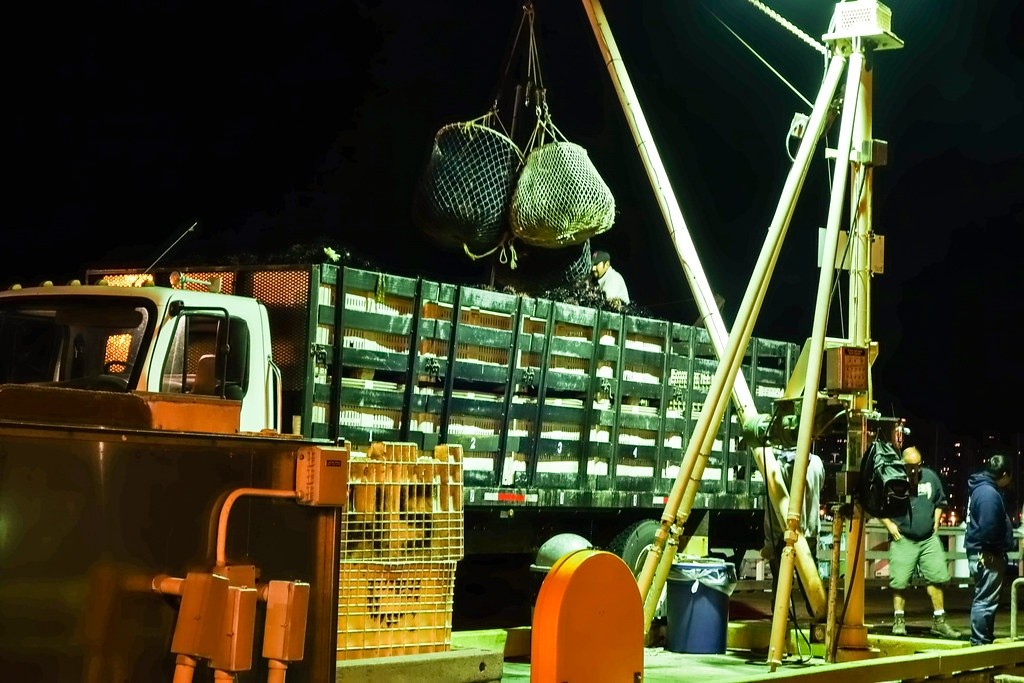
667 579 727 655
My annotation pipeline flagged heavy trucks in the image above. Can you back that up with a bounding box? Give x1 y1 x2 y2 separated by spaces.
0 248 800 625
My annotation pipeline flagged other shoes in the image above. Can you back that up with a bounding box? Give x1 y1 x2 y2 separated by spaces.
930 612 962 640
891 613 907 635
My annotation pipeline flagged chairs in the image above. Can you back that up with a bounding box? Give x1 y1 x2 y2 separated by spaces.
191 353 217 395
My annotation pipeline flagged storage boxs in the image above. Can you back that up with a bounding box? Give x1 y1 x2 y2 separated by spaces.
336 439 465 660
314 281 784 475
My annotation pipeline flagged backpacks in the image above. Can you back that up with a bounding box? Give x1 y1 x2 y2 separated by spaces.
856 428 913 529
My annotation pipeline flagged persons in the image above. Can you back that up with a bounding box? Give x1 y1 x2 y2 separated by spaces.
879 447 962 638
964 455 1014 646
752 446 825 658
591 251 630 306
